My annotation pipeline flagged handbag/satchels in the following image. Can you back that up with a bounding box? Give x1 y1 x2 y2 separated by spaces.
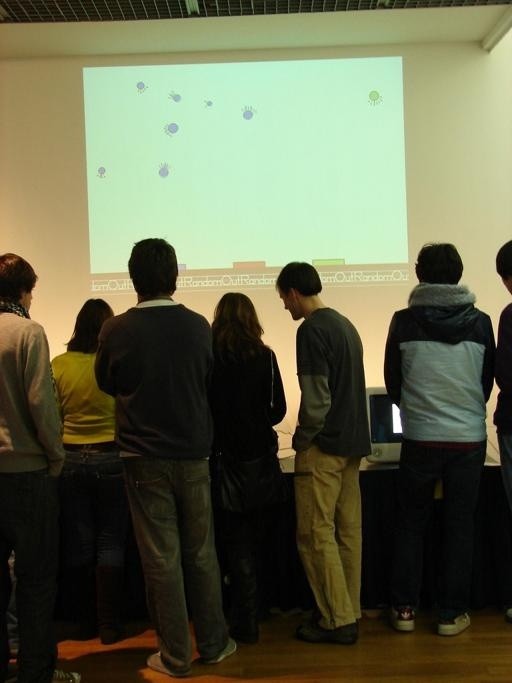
212 446 290 522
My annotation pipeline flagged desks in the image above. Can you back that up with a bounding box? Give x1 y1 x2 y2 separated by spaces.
269 456 504 612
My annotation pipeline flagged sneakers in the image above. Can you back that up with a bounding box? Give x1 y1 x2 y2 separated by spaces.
437 612 472 637
146 651 193 677
228 622 260 646
294 621 361 644
100 622 127 645
48 670 83 683
200 637 237 665
388 605 417 633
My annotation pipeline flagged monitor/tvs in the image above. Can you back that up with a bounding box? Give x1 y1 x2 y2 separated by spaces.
364 387 403 464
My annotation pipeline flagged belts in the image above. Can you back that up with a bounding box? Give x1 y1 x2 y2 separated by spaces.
63 440 116 454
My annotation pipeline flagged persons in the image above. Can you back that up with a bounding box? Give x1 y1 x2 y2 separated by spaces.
6 550 20 657
0 252 82 683
94 238 237 677
384 245 496 637
276 262 372 646
492 240 512 622
207 293 286 646
51 299 129 644
